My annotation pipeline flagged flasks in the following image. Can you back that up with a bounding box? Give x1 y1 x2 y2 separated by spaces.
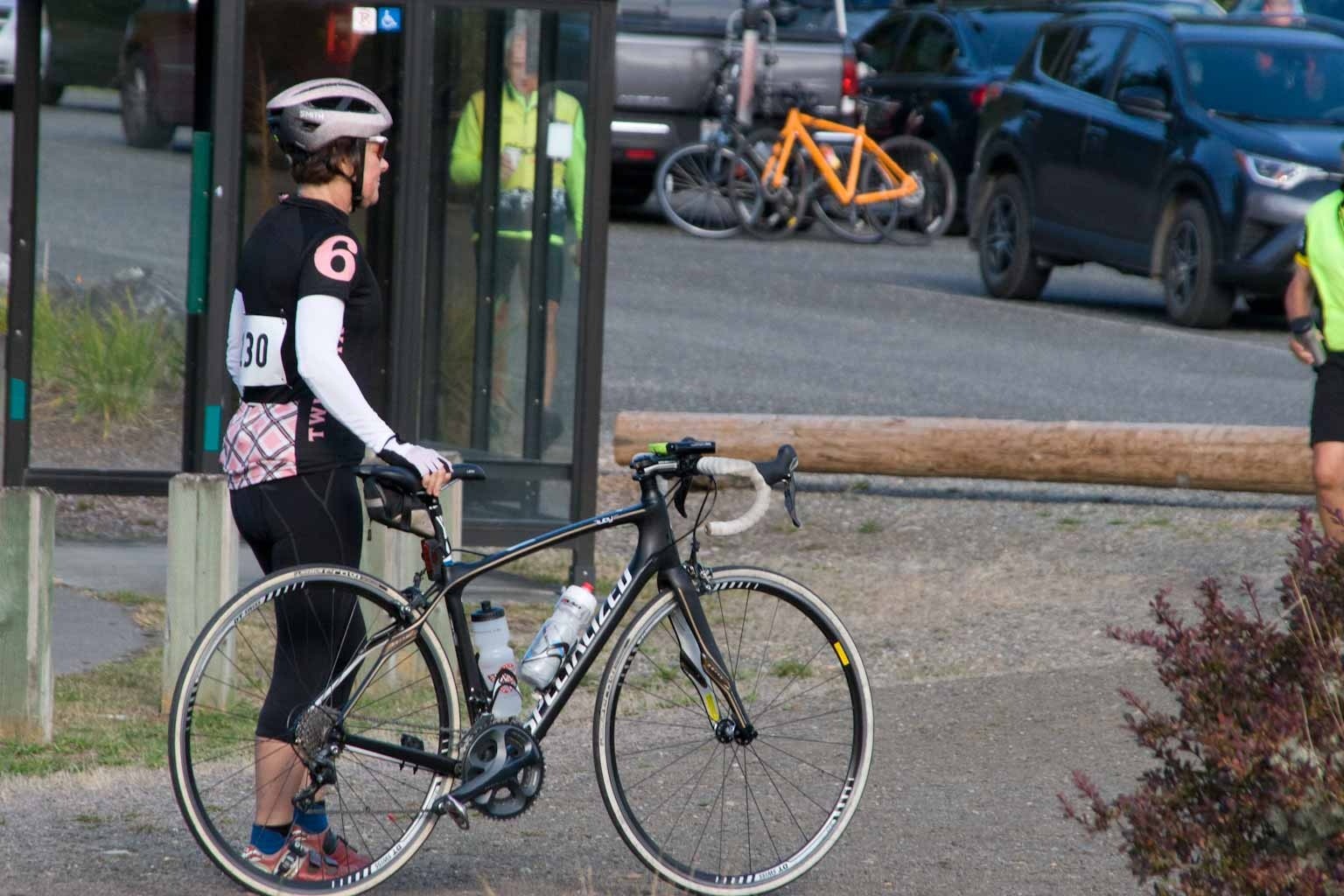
1291 315 1328 367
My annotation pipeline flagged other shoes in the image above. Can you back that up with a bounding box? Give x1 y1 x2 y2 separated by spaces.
541 412 564 450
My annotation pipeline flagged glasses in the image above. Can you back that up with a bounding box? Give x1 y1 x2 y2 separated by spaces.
367 136 388 161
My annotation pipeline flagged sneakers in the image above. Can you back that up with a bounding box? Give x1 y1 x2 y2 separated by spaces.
292 825 371 869
242 837 358 881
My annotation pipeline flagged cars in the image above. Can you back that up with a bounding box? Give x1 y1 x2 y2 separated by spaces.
1 0 66 113
119 0 378 154
962 0 1344 333
800 0 1344 240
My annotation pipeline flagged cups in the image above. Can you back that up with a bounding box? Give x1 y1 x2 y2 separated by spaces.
504 144 521 172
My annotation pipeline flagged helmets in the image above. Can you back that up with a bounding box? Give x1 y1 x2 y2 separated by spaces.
266 77 393 153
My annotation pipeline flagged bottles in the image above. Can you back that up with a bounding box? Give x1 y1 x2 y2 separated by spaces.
470 599 522 718
818 140 840 170
520 581 598 692
753 140 771 163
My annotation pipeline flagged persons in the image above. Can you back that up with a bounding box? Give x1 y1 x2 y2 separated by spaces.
449 22 585 453
1288 139 1343 547
218 81 452 882
1221 1 1316 114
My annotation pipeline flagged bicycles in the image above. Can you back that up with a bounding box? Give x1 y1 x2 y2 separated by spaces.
166 433 878 895
655 53 901 245
726 76 960 247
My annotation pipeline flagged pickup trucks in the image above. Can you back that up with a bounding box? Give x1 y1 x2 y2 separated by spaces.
606 1 861 223
